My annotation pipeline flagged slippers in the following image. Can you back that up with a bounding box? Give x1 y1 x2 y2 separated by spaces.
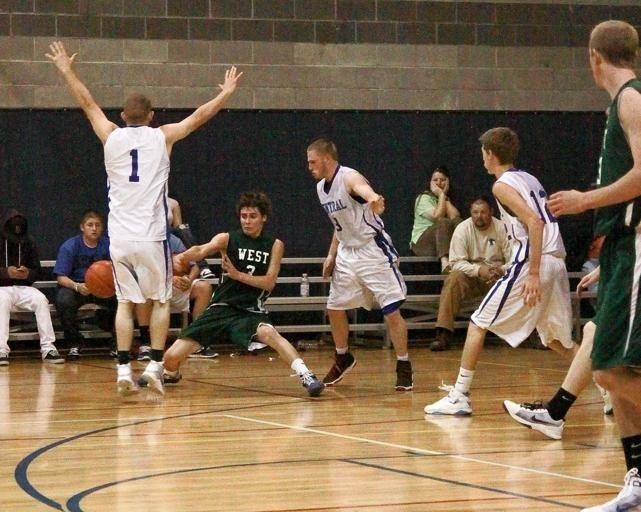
442 266 452 274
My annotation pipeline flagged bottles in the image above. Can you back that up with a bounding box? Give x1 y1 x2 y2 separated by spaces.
300 273 309 297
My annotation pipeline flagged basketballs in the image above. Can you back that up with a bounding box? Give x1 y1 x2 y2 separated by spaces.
84 260 116 299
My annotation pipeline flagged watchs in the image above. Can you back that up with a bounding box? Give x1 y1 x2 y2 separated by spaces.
75 283 79 292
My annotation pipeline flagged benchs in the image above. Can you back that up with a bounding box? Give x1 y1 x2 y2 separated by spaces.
10 255 596 359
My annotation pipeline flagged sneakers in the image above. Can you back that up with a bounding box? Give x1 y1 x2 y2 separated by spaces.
188 346 219 358
531 334 551 350
68 347 80 360
138 346 154 361
602 390 613 415
298 370 325 396
110 348 132 358
394 360 415 391
117 369 140 396
322 348 356 387
138 361 164 397
138 368 180 387
581 468 641 512
0 357 10 366
503 399 565 440
199 268 215 278
42 350 65 364
424 380 473 414
430 328 450 350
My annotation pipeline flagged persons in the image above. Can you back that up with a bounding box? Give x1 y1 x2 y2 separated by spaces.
428 195 511 352
422 128 614 416
137 233 211 362
53 210 133 360
166 196 215 279
45 41 243 397
503 264 613 440
409 166 463 275
0 210 64 365
547 21 640 512
583 243 601 304
306 138 413 391
161 192 325 398
